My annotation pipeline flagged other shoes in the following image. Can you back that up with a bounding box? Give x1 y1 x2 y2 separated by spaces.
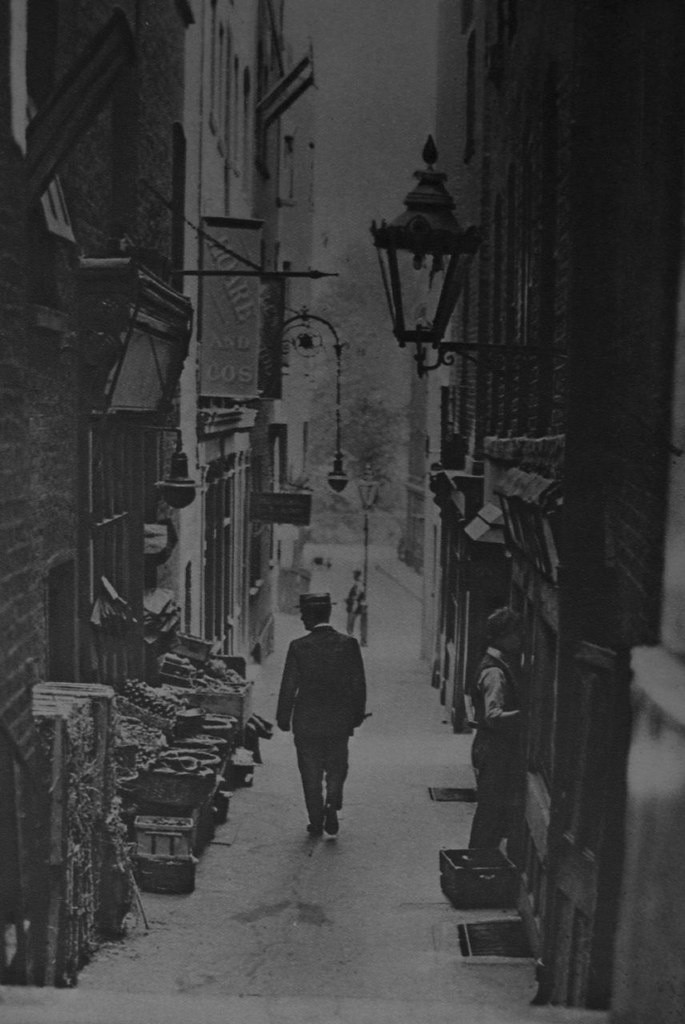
360 640 367 647
325 803 339 835
306 824 324 834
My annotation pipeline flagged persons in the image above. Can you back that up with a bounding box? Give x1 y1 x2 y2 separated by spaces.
345 567 365 633
468 607 529 850
276 595 365 840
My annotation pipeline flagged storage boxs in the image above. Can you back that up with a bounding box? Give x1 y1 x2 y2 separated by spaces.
130 815 197 894
190 681 253 729
439 848 519 910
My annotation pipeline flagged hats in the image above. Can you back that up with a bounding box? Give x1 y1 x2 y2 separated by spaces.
291 592 337 608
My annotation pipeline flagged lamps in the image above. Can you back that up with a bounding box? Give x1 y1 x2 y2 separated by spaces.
127 424 197 510
260 304 350 492
370 132 684 467
